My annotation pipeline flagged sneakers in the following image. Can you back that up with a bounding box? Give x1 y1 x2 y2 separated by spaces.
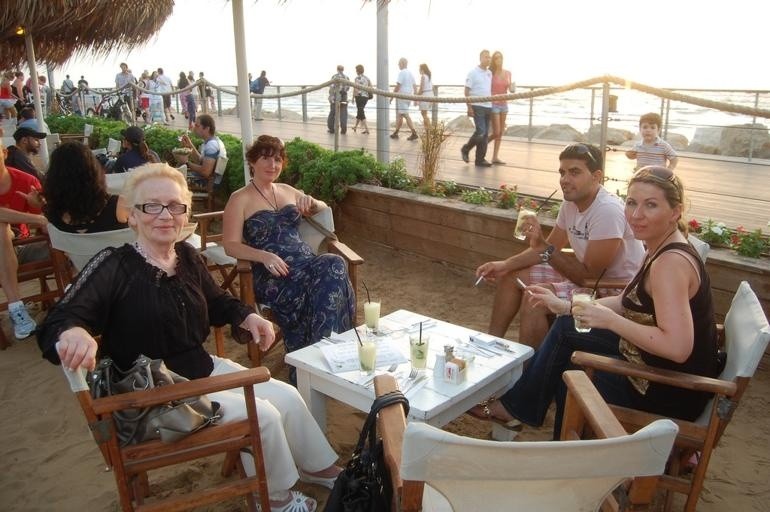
8 304 37 340
391 134 398 138
475 158 491 165
492 158 506 164
462 146 469 162
408 134 418 139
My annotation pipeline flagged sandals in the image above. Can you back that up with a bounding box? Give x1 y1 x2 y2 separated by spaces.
467 395 523 432
244 490 318 512
299 465 344 490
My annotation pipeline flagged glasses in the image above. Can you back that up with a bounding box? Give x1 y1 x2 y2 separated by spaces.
633 166 682 202
134 202 187 215
563 144 599 165
258 135 285 150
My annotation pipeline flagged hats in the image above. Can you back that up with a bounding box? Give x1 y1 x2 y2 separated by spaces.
120 126 146 143
14 126 47 141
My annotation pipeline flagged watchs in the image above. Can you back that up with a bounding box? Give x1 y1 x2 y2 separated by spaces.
539 246 555 263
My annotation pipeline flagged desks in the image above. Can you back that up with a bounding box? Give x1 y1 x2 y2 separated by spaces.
283 309 535 444
104 173 127 195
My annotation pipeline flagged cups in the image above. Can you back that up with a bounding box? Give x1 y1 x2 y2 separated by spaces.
571 287 598 334
362 297 382 332
356 337 377 376
408 335 430 371
509 83 516 93
512 204 540 241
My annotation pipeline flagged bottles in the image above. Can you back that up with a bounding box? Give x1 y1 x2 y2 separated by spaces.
444 345 455 363
434 353 446 380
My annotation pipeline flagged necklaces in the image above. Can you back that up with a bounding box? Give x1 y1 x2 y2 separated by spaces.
135 242 174 275
250 177 279 213
646 228 678 260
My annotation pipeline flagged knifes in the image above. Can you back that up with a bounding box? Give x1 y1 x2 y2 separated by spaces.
403 375 425 396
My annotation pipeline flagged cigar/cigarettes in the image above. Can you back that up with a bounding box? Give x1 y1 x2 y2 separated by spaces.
517 278 533 294
475 275 483 286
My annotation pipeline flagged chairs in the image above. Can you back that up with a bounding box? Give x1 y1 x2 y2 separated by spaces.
185 154 231 214
236 190 365 367
554 232 710 318
56 124 94 149
92 138 122 160
570 282 770 512
55 331 269 511
373 370 679 512
0 211 237 351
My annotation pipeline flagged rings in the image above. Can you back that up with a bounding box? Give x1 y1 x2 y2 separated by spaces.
268 265 272 268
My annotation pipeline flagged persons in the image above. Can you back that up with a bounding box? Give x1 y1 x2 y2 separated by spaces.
326 65 350 134
352 64 373 134
390 57 419 141
461 50 493 168
222 134 357 386
248 70 270 120
625 112 678 170
466 165 723 432
488 51 512 165
474 143 646 374
417 62 439 127
33 161 346 512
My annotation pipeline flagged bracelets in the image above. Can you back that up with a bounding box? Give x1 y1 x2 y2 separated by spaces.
568 301 573 315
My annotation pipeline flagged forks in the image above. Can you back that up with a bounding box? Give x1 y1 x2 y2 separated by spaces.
365 361 398 387
400 367 418 391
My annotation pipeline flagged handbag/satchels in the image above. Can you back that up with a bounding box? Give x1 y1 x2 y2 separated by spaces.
323 436 393 512
368 85 373 98
92 357 213 444
